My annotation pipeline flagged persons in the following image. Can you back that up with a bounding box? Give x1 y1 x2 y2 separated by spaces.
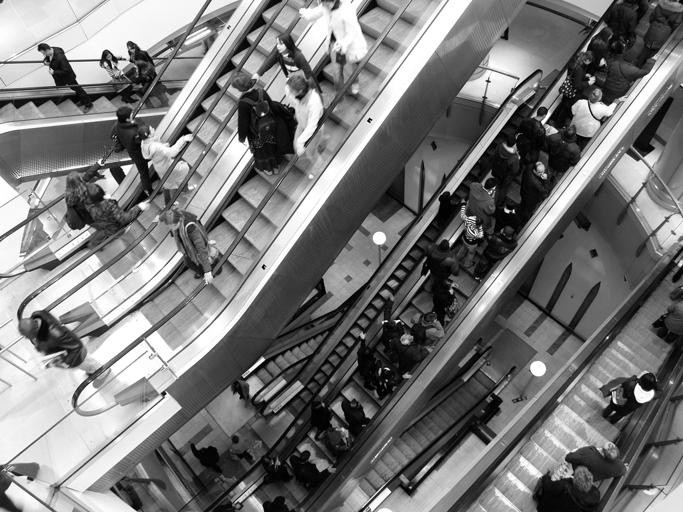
132 52 169 110
132 126 198 210
0 460 41 511
157 209 222 286
99 49 139 104
229 0 682 511
108 107 162 195
17 310 112 387
63 156 118 251
125 42 162 98
82 183 152 259
35 42 94 116
187 442 223 475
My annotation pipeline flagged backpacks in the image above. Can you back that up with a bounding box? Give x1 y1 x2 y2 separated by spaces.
410 322 436 347
63 195 85 230
240 89 276 138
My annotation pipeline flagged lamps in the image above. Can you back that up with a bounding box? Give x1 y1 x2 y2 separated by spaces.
372 231 386 264
512 360 547 402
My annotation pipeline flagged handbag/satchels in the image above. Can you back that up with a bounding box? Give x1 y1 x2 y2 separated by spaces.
113 136 126 153
599 376 627 407
651 312 670 328
193 242 219 267
559 76 578 99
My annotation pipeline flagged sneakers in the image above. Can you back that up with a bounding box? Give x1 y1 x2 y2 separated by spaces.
253 164 319 177
93 367 112 388
24 462 39 486
350 80 359 96
75 100 93 113
120 95 170 108
144 182 198 208
193 272 203 280
85 242 147 262
